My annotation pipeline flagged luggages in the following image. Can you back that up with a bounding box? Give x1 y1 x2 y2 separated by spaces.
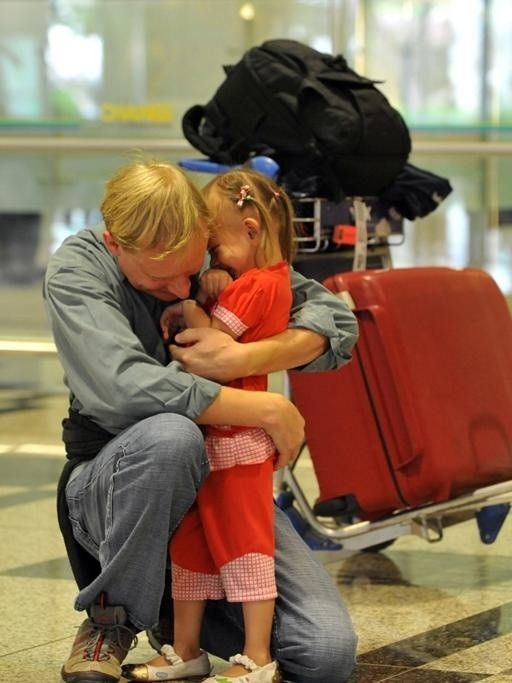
283 262 511 524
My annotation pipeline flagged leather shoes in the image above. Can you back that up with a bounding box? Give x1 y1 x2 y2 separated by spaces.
120 643 212 683
200 654 284 683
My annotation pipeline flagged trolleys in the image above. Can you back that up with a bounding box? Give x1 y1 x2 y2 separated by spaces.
173 39 510 556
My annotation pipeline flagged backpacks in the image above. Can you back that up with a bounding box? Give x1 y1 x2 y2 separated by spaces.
181 35 414 216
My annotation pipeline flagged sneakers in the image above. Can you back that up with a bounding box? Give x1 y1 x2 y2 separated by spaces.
59 600 137 683
145 616 175 656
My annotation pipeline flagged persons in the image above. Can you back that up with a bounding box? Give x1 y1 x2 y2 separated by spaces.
45 155 357 683
120 169 294 682
